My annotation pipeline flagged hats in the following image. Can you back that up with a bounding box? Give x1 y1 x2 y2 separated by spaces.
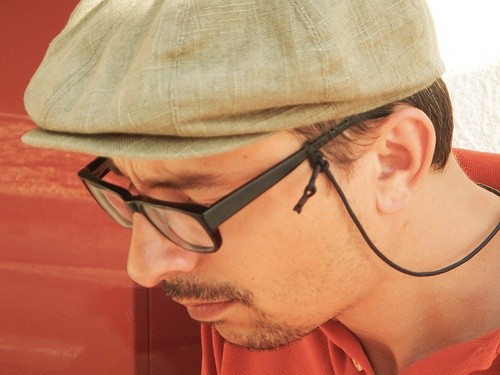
20 0 443 159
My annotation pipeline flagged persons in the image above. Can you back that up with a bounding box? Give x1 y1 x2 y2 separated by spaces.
48 1 499 374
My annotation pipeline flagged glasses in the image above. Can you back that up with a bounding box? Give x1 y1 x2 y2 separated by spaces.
76 107 394 254
